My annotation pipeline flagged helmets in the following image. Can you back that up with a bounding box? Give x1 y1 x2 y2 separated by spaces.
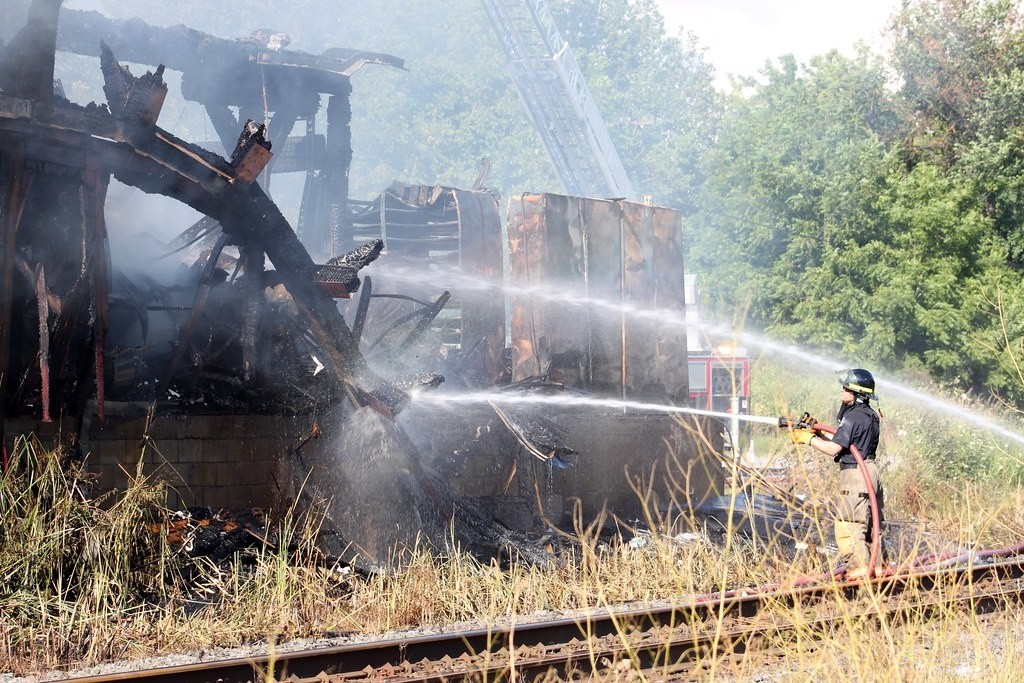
836 369 879 401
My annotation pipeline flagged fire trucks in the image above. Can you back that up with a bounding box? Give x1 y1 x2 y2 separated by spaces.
483 0 792 499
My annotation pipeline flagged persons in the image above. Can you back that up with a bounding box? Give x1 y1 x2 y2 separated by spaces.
792 370 880 573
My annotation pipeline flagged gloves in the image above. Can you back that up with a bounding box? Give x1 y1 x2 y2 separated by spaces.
791 428 817 446
810 427 826 437
